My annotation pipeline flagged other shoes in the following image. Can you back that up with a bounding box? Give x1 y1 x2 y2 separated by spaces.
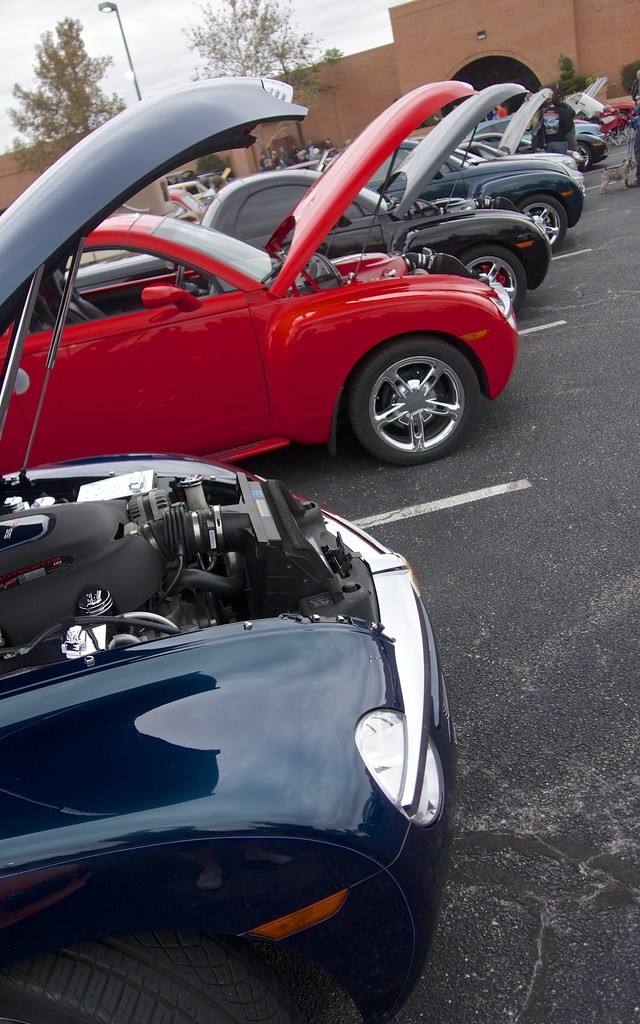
628 179 640 187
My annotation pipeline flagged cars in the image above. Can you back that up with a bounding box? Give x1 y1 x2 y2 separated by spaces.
0 76 635 320
0 80 518 486
0 73 454 1024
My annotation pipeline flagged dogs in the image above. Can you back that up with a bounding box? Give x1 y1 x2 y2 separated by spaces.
600 156 637 194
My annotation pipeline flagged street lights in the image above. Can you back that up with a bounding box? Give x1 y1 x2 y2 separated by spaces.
97 1 169 199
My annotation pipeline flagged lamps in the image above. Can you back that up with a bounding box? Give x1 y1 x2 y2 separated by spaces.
97 0 119 17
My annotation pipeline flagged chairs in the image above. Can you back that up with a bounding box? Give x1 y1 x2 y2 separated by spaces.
600 117 634 146
55 272 106 323
6 291 56 333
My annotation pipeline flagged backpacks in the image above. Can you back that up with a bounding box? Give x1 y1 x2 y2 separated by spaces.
531 111 546 149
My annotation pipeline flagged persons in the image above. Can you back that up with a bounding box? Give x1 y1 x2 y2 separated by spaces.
492 103 507 119
259 137 351 171
552 81 565 99
524 91 576 153
432 113 442 123
629 70 640 187
199 175 228 193
